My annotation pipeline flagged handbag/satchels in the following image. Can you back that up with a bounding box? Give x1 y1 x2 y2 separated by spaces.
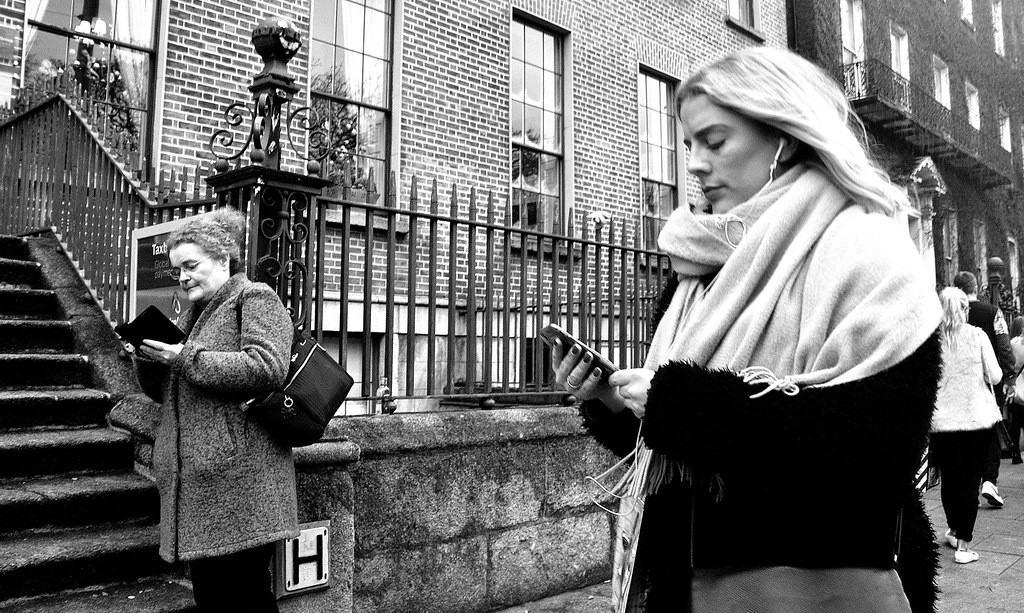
1013 375 1024 405
234 287 355 447
690 563 911 612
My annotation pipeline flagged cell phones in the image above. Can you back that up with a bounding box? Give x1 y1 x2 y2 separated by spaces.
540 323 622 380
113 305 186 355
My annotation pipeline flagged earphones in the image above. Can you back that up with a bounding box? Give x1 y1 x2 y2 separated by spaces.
774 136 788 161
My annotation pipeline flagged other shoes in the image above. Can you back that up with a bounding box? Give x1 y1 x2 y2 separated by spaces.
945 528 958 548
1012 452 1023 464
954 550 980 563
981 481 1004 506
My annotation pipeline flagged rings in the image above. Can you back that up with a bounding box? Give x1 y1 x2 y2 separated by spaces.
566 377 582 389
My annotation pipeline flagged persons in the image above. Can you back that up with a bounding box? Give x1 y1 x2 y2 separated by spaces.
551 43 940 613
134 206 301 613
929 271 1024 564
